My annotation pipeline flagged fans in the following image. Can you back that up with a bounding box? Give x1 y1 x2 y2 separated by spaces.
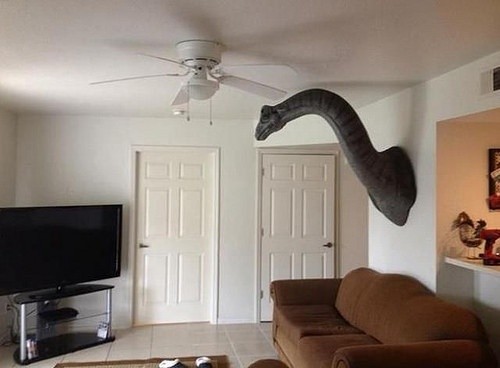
88 39 300 107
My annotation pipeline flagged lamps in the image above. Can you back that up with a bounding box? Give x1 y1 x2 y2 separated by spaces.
180 59 221 101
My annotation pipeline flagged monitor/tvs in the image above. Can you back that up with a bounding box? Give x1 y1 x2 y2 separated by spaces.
0 204 123 296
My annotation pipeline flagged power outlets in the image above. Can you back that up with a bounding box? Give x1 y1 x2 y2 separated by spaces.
5 303 10 313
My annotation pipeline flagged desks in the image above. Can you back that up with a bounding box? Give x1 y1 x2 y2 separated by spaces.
13 284 116 366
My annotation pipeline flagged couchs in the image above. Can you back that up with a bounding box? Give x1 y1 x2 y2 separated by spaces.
269 267 498 368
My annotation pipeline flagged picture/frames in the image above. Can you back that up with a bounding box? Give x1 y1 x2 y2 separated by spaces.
487 147 500 212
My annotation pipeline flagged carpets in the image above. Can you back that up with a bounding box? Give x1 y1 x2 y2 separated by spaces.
54 355 230 368
